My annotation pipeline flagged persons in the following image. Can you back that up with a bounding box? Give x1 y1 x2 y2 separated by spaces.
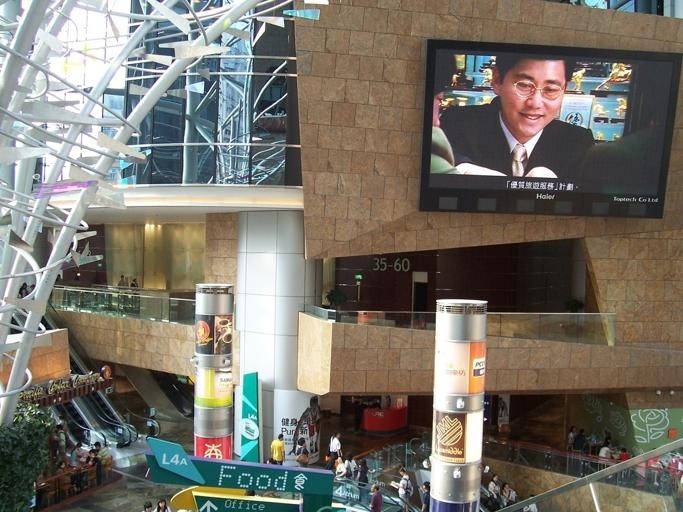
33 440 116 512
573 58 672 193
15 265 149 312
422 453 432 471
270 433 286 466
567 425 680 495
325 431 369 502
153 498 170 512
487 474 538 511
141 501 154 512
369 483 382 511
431 48 458 175
295 437 309 467
437 52 596 186
419 481 430 511
393 467 411 505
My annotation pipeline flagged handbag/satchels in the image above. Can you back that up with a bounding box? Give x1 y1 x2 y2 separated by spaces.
296 454 309 464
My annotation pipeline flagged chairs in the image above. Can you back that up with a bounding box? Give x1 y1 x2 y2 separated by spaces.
35 454 113 508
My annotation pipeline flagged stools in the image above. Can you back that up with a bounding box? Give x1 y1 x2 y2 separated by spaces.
565 443 679 492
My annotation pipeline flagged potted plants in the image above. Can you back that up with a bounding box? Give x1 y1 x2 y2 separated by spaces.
563 299 585 336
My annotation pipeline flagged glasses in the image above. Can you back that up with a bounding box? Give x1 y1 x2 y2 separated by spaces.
512 80 563 99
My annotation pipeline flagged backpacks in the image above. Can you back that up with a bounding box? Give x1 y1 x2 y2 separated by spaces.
403 476 414 497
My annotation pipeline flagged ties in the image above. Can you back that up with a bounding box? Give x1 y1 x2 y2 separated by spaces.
512 146 526 177
